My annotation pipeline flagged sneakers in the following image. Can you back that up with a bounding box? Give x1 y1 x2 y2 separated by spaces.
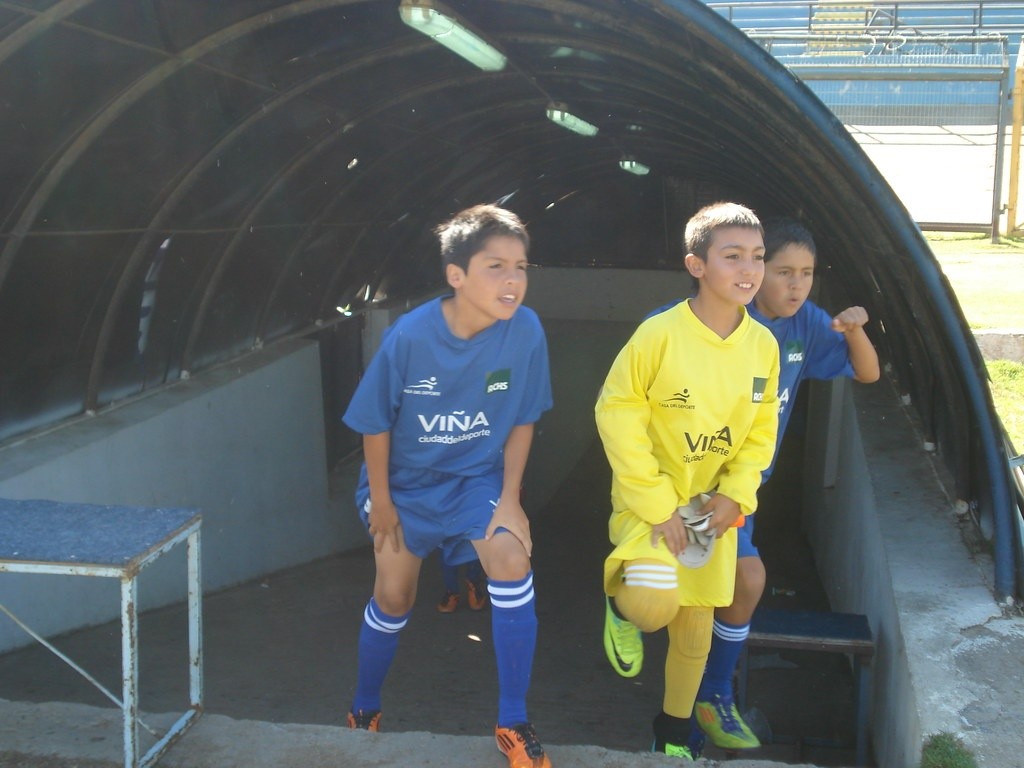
347 710 382 732
694 690 762 753
436 592 460 614
495 723 553 768
603 594 643 678
650 711 698 762
467 577 487 611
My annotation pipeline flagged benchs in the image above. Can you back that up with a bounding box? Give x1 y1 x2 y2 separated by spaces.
701 1 1024 58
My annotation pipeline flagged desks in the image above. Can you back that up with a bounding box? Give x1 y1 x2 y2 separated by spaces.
0 497 205 768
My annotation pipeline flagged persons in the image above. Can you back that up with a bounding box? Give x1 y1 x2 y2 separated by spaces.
342 205 554 768
597 202 880 760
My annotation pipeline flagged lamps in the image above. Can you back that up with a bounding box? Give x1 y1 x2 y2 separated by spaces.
397 0 653 177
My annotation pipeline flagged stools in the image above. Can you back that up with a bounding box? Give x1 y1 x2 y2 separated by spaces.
738 614 874 768
